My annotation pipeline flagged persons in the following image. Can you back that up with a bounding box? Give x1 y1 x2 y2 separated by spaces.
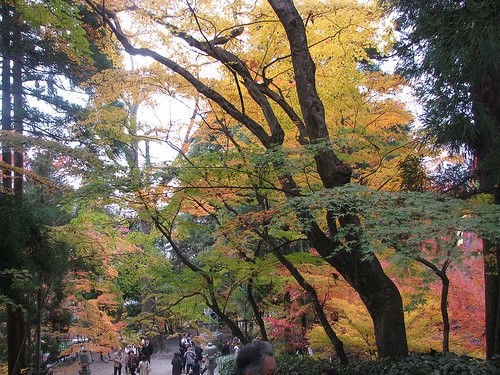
113 348 124 375
200 367 207 375
178 332 203 361
125 344 132 375
131 338 153 356
192 358 200 375
171 351 182 375
236 341 276 375
138 355 150 375
221 340 238 355
183 347 195 375
127 353 139 375
